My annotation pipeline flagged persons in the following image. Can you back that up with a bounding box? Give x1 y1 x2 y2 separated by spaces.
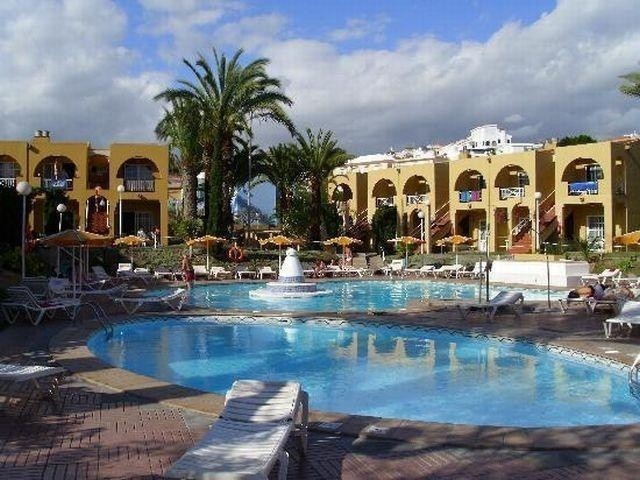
606 282 640 312
136 226 147 245
567 282 612 298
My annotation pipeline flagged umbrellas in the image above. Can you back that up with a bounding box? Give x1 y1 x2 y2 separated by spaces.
258 235 298 276
386 235 426 269
323 236 364 269
435 240 447 253
441 235 473 252
186 234 226 271
35 227 115 301
612 230 640 247
112 234 151 270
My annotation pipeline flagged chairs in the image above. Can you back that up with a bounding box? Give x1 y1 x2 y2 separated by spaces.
558 268 640 340
1 260 187 325
302 265 375 278
457 289 525 324
378 259 487 280
256 266 278 280
210 265 231 280
0 363 67 416
159 380 309 480
192 263 209 281
234 265 256 281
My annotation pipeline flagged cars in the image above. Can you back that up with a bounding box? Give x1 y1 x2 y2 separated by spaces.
118 262 258 284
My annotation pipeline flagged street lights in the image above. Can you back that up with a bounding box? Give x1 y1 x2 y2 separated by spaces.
534 192 542 252
57 204 67 277
541 241 559 311
16 181 32 279
117 185 125 237
418 211 425 253
